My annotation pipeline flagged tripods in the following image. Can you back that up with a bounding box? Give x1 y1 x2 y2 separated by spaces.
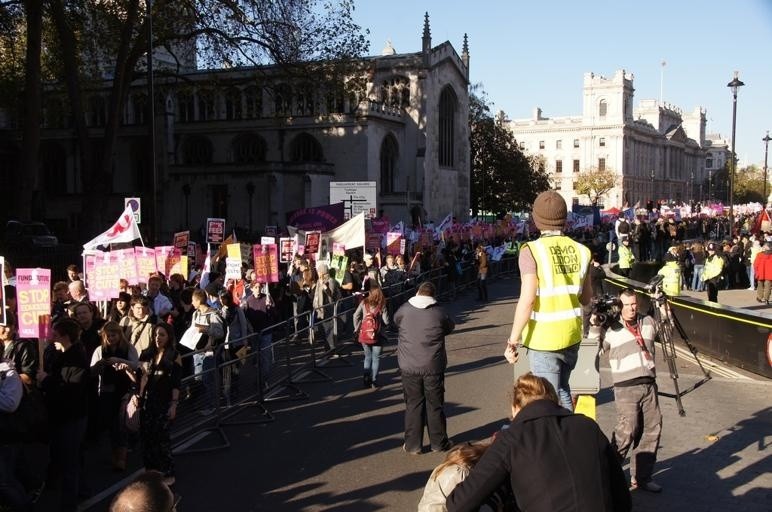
644 273 710 416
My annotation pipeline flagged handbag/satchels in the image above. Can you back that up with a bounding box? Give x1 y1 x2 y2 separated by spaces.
121 392 141 432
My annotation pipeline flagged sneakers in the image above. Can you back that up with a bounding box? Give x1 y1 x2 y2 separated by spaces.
644 482 662 493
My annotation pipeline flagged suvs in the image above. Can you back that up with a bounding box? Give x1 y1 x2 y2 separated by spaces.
4 217 59 248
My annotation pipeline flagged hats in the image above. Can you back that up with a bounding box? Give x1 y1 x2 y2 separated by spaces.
708 242 717 251
621 237 630 242
663 252 674 260
532 191 567 230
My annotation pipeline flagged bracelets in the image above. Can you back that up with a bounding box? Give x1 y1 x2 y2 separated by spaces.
506 338 518 353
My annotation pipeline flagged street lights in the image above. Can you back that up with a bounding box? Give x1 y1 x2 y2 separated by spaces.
725 69 745 241
644 165 731 200
764 128 770 210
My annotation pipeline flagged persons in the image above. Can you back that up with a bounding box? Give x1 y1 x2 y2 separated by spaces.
353 216 535 303
417 429 513 512
588 284 675 493
352 285 391 393
618 237 635 278
111 472 176 512
657 230 772 305
1 258 138 507
598 201 772 264
446 373 633 512
122 257 351 475
504 190 592 419
393 281 457 455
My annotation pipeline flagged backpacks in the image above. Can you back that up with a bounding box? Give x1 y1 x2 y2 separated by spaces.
357 299 384 345
194 312 227 344
618 218 630 234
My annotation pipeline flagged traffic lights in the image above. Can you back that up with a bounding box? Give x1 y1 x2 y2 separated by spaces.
691 200 697 212
697 204 701 213
646 201 654 212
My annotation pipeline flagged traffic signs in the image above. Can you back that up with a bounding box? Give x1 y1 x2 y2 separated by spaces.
329 180 376 222
